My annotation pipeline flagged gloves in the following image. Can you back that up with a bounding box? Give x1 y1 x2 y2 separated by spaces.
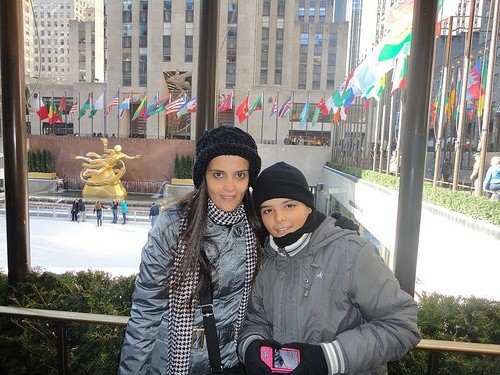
245 339 329 375
331 211 359 230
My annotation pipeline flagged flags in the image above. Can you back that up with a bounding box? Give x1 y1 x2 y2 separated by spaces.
34 0 443 128
430 53 491 123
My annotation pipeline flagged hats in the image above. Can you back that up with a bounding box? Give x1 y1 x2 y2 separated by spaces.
193 125 262 189
152 203 155 205
252 162 316 210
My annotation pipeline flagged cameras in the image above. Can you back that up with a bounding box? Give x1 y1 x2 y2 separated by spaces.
260 347 301 374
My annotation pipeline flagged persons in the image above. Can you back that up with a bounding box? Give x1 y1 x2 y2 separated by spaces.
75 137 139 176
240 162 421 375
149 202 159 227
71 199 86 222
469 153 486 193
93 201 109 227
482 157 500 197
431 158 453 182
111 198 118 223
120 200 128 224
390 150 402 176
119 127 360 375
